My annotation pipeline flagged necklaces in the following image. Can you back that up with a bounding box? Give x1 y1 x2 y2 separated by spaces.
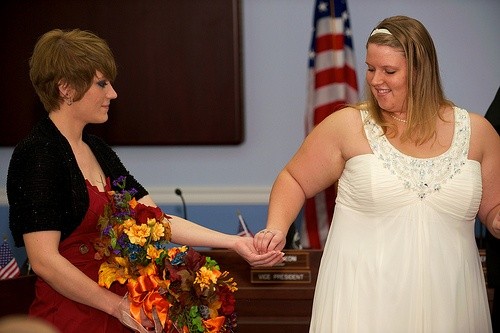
389 104 440 123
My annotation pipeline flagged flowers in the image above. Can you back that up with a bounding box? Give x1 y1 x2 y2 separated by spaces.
93 175 237 333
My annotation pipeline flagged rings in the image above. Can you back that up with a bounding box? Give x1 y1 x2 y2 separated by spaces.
265 231 275 237
259 230 266 234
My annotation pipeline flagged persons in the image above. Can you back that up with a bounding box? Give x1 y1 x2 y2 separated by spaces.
7 26 286 333
253 17 500 333
483 87 500 333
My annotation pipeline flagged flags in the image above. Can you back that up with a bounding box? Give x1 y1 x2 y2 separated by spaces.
300 0 359 251
237 218 255 238
0 240 21 280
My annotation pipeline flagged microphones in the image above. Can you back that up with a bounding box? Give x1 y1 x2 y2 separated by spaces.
175 188 186 219
